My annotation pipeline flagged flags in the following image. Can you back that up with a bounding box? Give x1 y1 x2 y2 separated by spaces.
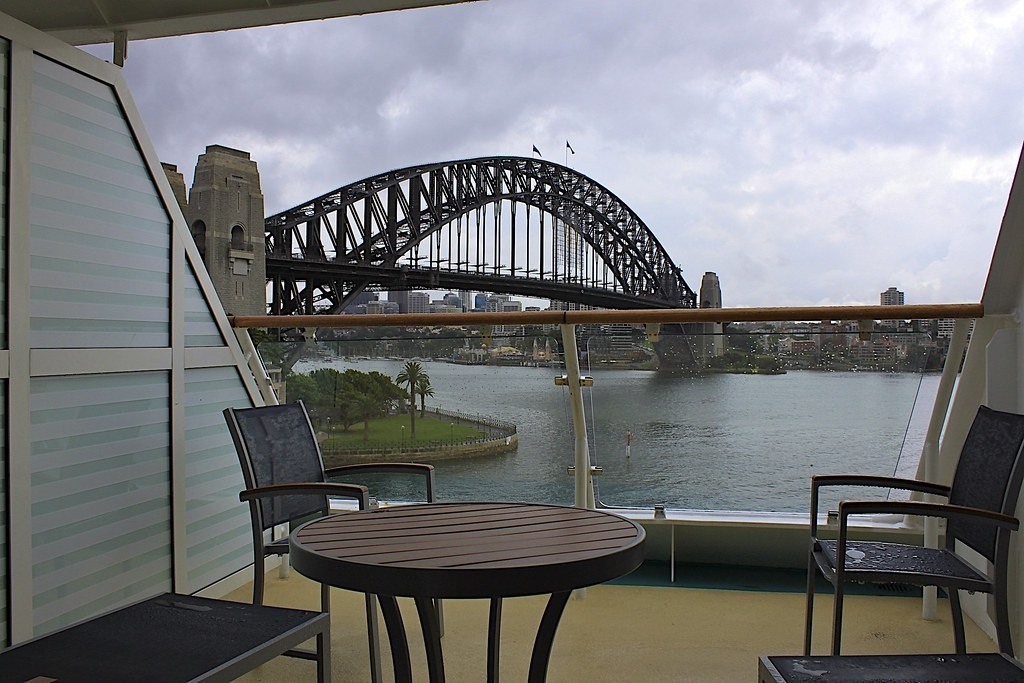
533 145 542 156
567 141 574 153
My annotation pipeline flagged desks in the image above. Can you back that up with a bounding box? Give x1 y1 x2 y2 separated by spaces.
756 653 1024 683
290 501 645 683
0 593 333 683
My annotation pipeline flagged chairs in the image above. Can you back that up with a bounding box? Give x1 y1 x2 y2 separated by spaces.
221 401 435 600
804 405 1024 656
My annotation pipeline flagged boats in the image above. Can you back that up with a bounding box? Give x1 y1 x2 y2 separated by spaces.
298 353 432 366
